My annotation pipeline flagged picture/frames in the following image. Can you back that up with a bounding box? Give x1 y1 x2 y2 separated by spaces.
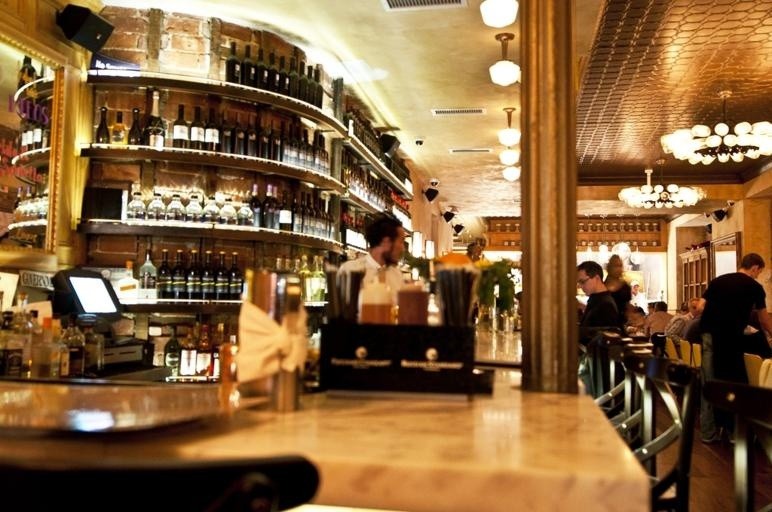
710 231 741 278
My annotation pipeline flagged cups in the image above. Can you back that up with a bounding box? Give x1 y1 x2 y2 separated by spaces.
361 280 431 325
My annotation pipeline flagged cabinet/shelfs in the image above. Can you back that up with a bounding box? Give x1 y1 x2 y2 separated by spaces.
485 218 665 252
6 65 50 245
677 243 738 305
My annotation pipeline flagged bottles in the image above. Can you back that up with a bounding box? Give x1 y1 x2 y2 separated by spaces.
0 290 101 382
93 40 333 176
7 49 52 246
120 178 339 303
341 103 415 262
494 221 662 246
162 319 238 384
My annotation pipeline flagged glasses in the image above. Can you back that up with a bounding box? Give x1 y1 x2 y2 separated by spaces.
580 277 590 284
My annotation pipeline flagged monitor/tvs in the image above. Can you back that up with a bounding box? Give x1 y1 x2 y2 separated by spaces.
46 269 122 323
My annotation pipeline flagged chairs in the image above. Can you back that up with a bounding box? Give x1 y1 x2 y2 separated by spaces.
577 321 770 511
0 447 323 512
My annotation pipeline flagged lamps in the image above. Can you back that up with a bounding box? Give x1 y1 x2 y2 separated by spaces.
617 158 701 209
480 0 525 184
658 88 771 165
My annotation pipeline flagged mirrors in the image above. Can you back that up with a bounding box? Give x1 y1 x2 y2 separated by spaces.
0 11 68 254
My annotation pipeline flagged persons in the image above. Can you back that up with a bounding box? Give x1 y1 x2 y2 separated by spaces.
624 296 705 341
333 211 405 329
604 254 632 320
693 251 772 446
577 258 618 354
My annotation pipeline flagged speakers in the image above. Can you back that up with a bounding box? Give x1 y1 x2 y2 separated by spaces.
454 224 465 234
710 210 727 223
57 4 115 54
443 211 455 223
378 132 401 158
424 188 440 202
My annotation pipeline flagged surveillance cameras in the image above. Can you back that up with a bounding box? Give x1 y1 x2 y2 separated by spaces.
429 178 440 186
416 140 424 147
727 200 736 207
704 211 711 218
